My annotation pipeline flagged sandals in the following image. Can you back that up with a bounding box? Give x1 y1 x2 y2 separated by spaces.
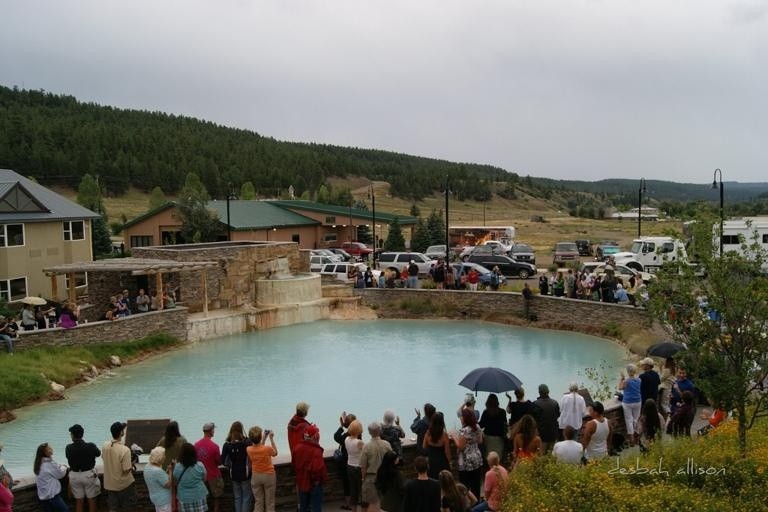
341 505 352 510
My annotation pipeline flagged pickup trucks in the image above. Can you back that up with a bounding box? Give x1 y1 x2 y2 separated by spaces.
423 245 453 263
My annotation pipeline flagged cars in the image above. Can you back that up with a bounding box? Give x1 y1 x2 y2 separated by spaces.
450 261 506 290
310 256 337 273
310 248 352 262
363 243 383 258
459 241 535 264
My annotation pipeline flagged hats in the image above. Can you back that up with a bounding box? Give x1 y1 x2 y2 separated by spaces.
202 423 214 431
110 422 126 433
296 402 310 411
303 425 319 437
68 424 83 435
639 358 653 366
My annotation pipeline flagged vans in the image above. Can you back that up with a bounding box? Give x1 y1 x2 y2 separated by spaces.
468 255 537 280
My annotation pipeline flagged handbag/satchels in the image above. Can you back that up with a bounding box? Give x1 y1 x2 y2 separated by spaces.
333 445 343 460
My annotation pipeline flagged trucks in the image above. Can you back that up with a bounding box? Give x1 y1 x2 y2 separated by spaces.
614 215 768 279
342 242 378 260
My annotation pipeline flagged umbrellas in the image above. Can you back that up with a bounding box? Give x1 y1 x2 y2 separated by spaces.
646 341 684 359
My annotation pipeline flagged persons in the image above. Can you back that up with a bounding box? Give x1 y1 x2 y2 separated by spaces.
346 255 654 321
660 288 767 355
0 356 768 509
0 274 186 354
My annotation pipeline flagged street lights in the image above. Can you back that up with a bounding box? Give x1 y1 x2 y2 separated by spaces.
226 181 237 241
638 176 646 236
711 167 724 264
367 184 376 267
444 172 450 266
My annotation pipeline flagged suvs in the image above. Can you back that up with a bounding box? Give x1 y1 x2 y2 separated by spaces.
575 239 595 256
552 242 580 265
375 252 446 279
322 262 381 284
595 242 622 262
576 262 657 288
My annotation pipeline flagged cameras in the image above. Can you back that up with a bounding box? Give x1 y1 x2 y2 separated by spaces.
265 430 270 434
